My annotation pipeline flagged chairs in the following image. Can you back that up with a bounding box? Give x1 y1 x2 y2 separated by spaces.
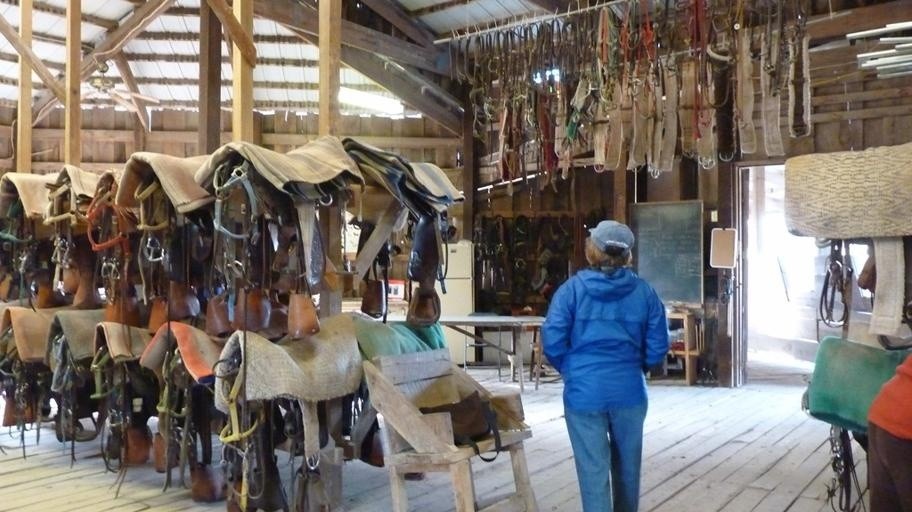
361 347 540 512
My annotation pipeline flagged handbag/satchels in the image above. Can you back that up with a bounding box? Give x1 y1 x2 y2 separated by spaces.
417 372 502 463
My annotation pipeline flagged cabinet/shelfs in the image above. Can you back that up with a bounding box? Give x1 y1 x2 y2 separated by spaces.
648 312 700 387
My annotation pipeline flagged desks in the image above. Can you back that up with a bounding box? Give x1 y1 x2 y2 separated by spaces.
377 310 547 394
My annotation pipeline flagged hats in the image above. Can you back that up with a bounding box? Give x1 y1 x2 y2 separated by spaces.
587 217 636 259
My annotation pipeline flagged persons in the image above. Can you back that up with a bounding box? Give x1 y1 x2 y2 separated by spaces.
865 352 912 511
537 218 672 512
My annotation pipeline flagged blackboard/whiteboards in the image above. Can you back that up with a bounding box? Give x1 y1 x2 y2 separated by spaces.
628 200 704 308
